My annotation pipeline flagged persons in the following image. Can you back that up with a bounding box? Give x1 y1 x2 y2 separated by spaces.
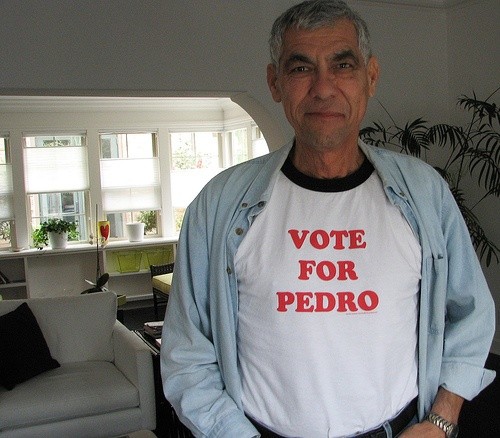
160 0 496 438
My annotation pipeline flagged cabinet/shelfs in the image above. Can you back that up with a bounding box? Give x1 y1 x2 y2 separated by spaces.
0 242 177 302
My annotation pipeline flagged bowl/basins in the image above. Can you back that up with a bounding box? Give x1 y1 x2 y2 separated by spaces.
111 250 144 274
142 247 171 270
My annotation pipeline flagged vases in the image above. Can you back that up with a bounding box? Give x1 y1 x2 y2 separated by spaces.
126 222 146 242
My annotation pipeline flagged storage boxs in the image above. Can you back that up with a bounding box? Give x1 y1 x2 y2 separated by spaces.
141 246 172 269
112 250 142 273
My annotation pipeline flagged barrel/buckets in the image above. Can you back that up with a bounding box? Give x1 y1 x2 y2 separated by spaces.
126 223 145 242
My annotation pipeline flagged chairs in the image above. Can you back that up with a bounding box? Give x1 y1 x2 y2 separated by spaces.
150 263 175 321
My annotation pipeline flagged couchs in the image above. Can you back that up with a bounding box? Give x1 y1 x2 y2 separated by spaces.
0 291 157 438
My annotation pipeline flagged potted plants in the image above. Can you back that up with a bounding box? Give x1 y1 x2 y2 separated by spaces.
359 87 500 438
32 218 80 250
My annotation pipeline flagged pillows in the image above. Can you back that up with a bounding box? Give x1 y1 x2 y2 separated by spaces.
0 302 60 391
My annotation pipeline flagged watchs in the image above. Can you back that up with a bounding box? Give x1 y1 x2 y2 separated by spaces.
426 411 459 438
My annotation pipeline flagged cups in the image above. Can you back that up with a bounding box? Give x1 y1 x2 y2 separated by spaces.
98 221 110 245
